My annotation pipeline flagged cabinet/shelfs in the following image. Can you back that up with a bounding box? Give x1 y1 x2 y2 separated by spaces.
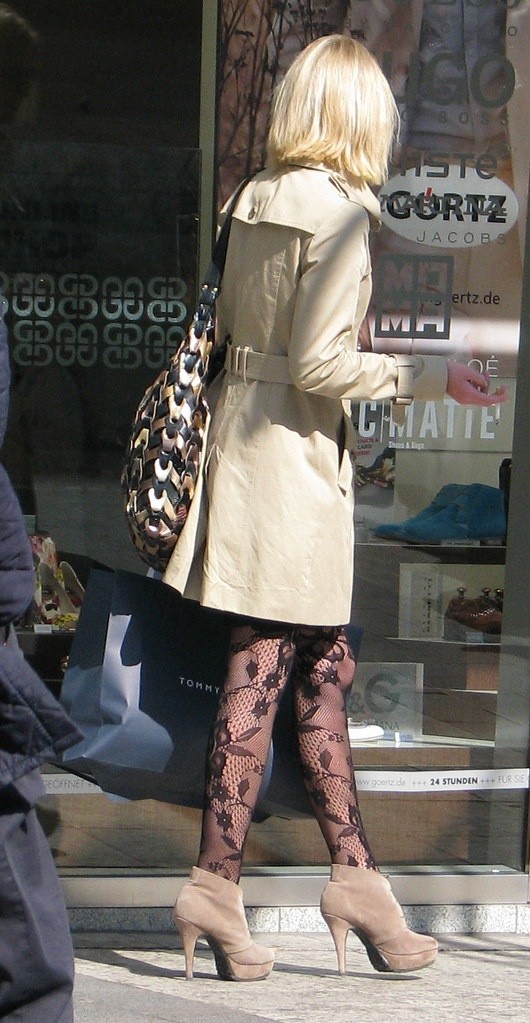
352 543 508 742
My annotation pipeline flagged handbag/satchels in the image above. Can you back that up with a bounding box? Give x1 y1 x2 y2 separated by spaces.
120 166 269 573
46 566 365 821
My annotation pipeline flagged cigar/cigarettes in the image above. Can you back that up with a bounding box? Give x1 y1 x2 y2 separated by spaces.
495 387 500 424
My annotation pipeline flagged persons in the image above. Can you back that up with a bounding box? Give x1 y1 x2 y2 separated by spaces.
176 35 509 979
0 294 77 1023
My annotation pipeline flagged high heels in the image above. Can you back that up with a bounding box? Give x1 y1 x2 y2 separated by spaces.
321 863 438 975
173 865 275 981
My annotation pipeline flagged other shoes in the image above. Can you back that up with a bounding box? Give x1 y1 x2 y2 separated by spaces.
443 598 503 642
372 482 468 541
404 483 507 546
